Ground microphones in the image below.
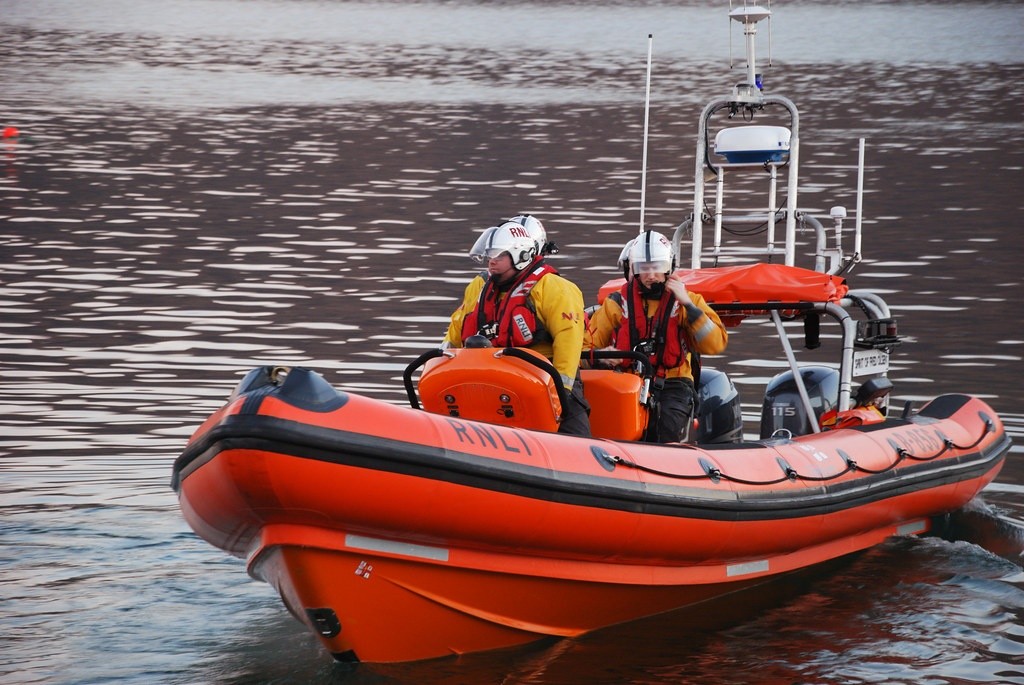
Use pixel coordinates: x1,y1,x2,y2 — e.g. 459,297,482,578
491,259,522,282
650,281,667,294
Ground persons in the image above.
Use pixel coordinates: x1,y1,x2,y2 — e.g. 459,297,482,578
581,229,729,445
440,213,592,440
616,238,638,283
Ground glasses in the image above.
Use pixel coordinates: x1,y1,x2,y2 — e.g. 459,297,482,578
486,256,502,260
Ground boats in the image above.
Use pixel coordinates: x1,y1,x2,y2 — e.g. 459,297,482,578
172,0,1013,662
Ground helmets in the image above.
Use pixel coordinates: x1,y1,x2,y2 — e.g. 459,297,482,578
621,231,676,275
485,215,548,270
